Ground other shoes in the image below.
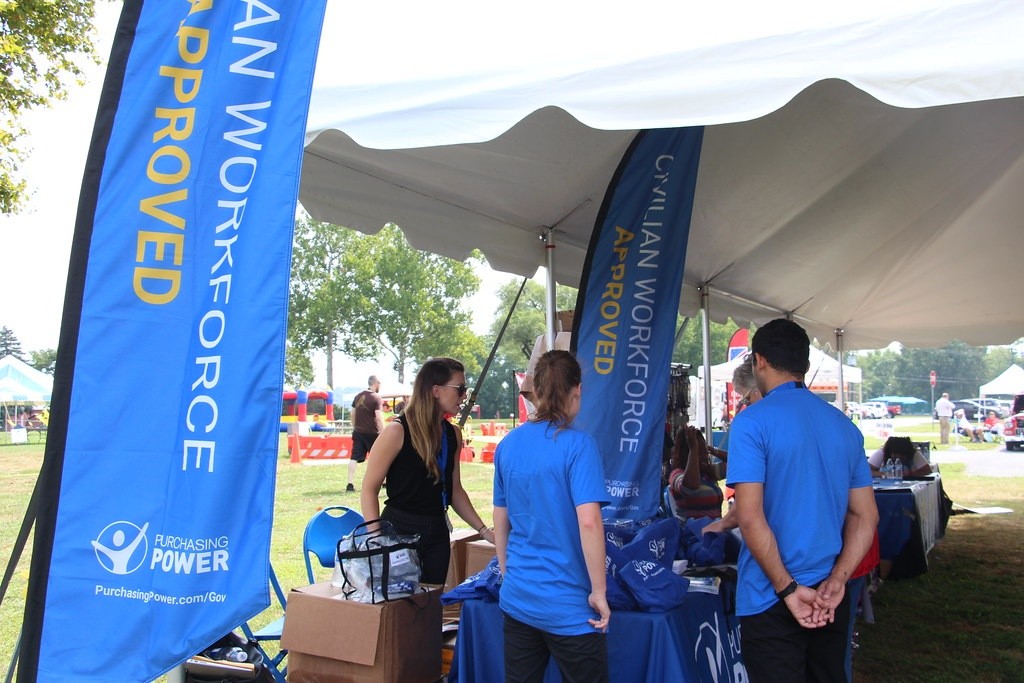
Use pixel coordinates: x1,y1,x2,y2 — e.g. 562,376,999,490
383,483,386,488
346,483,353,491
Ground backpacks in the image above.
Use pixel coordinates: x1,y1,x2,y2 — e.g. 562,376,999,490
664,485,686,522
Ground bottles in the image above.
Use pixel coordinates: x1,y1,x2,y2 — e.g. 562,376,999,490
885,458,894,478
204,647,248,664
894,458,903,480
332,535,352,587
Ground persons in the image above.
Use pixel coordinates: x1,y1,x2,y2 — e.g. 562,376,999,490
668,318,879,683
310,414,326,431
935,393,956,444
984,411,1002,429
361,358,495,593
954,409,985,443
492,348,612,683
21,410,36,427
346,374,387,491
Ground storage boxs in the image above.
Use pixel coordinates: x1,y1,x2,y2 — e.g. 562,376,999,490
442,528,496,620
280,582,445,682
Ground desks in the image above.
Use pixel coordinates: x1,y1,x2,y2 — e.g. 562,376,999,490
445,578,751,683
872,472,943,579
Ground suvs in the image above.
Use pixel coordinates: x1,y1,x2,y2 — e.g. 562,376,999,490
828,401,901,420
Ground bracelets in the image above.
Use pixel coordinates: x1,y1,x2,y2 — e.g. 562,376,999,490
478,525,486,532
774,578,799,601
480,528,489,537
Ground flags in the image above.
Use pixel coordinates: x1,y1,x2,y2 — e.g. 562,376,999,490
15,0,325,682
568,126,705,521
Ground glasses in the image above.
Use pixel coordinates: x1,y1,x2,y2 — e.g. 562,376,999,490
445,384,468,397
738,388,751,405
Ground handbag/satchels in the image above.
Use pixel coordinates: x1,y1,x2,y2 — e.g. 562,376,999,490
184,632,275,683
331,518,424,604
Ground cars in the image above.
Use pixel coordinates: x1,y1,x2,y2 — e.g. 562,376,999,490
1003,391,1024,451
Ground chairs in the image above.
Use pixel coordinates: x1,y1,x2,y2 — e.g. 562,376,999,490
303,505,366,584
664,484,685,521
242,562,288,683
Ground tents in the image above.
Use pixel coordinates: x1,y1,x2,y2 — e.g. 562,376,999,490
977,363,1024,427
295,0,1024,448
695,342,862,433
0,353,54,443
